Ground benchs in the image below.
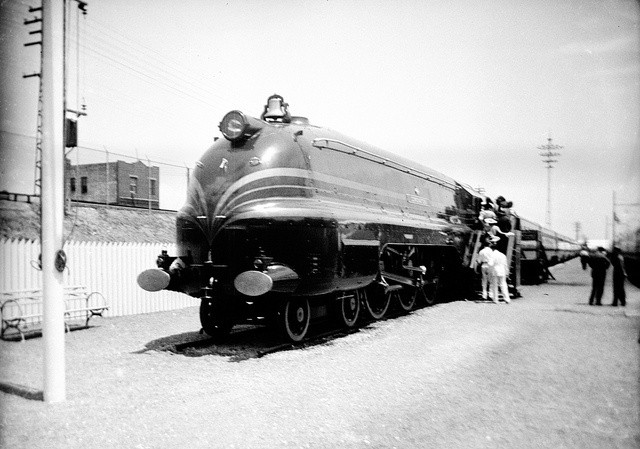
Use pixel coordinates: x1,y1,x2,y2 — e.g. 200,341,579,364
0,286,109,343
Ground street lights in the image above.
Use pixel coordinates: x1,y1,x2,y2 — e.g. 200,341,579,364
536,137,565,230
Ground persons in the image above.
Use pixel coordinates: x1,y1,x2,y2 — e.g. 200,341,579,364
475,197,512,248
478,243,493,300
588,247,611,305
489,246,511,304
580,247,589,269
611,246,627,305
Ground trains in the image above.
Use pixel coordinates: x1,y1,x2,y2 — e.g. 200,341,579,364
136,94,583,346
